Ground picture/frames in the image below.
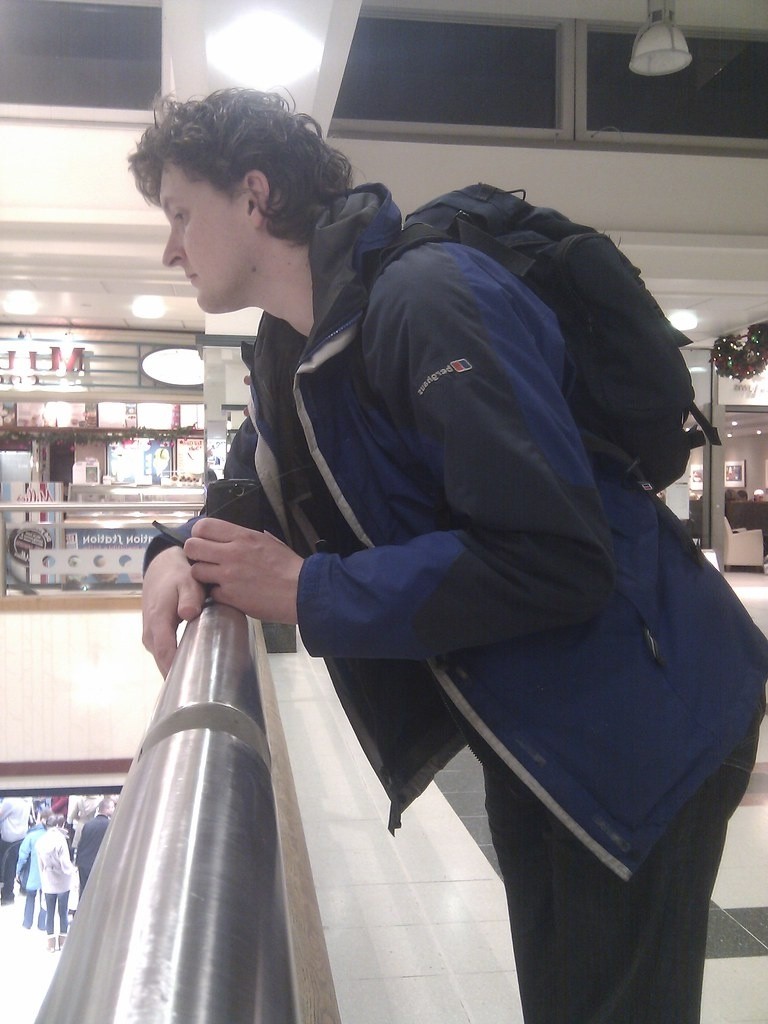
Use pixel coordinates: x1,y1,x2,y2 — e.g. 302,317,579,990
725,460,745,488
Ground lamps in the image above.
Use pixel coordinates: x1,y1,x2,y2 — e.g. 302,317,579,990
628,0,692,77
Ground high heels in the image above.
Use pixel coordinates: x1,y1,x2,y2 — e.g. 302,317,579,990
58,936,66,951
49,937,56,953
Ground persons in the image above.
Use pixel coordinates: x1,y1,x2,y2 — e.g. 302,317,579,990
129,88,768,1024
690,489,765,502
1,794,121,953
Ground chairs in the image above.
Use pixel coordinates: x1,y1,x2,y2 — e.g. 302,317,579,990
723,516,764,572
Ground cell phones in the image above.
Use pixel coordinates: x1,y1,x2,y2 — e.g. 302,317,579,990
205,478,264,533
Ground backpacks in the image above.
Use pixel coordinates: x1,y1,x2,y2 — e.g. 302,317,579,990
360,181,721,494
